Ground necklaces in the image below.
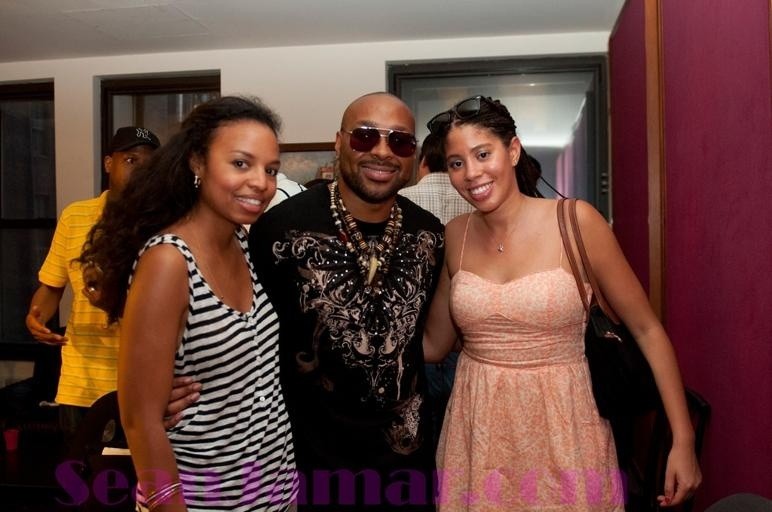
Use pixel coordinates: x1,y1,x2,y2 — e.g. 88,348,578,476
326,180,403,295
474,203,523,255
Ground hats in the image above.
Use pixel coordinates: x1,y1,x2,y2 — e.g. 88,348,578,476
109,126,161,154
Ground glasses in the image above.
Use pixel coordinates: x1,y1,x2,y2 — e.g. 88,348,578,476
341,127,418,157
427,94,485,135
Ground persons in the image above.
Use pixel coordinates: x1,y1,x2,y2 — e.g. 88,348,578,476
24,126,162,410
242,171,310,235
421,92,705,512
161,89,446,512
67,90,301,512
524,153,545,198
397,132,476,225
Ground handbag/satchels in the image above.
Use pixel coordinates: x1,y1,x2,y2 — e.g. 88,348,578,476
556,196,711,509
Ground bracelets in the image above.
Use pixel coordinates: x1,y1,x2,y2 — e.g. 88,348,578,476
143,482,183,511
